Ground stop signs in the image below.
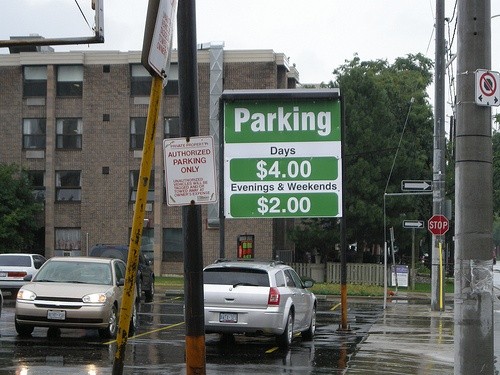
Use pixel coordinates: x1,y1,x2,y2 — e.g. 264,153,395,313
428,215,450,236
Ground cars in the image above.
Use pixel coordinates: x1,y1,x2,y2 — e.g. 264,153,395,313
14,256,139,340
0,253,48,289
183,258,318,349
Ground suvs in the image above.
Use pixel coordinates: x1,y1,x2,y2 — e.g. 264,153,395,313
88,242,155,300
